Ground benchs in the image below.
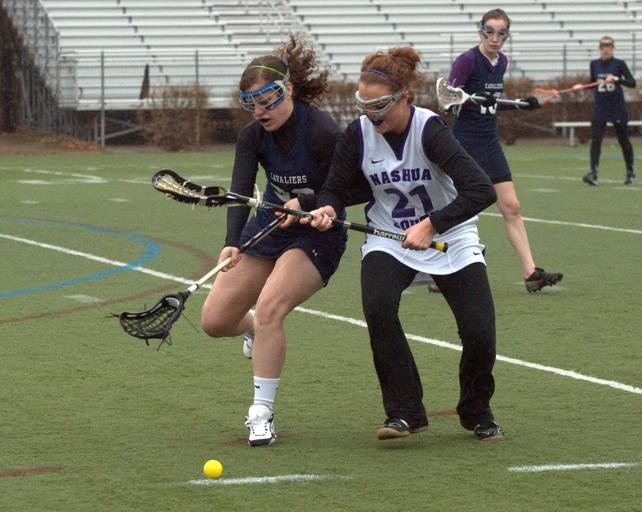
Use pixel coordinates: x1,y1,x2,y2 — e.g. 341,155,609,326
550,120,642,147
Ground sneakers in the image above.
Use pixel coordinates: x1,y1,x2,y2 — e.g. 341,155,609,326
624,174,635,186
244,404,277,447
428,284,439,293
474,422,504,441
243,308,255,360
376,416,429,439
526,268,563,292
583,172,600,185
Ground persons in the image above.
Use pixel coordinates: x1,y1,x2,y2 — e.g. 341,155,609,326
299,47,504,440
202,32,348,447
573,35,637,186
428,9,563,294
